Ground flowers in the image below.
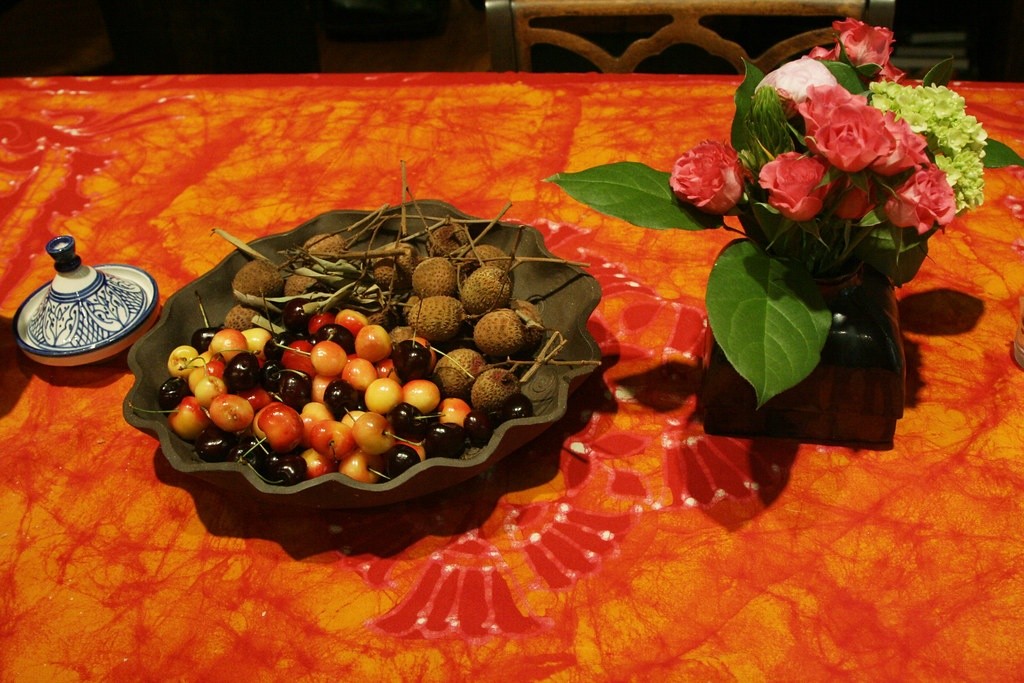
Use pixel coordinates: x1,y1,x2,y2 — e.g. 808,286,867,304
539,15,1024,410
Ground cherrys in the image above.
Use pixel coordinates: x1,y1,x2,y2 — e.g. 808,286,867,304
127,285,534,487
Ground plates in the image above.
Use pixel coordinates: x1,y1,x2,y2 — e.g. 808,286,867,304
11,263,161,366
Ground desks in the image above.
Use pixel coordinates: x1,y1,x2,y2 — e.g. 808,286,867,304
1,72,1024,683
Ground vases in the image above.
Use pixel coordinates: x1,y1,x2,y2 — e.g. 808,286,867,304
701,258,907,443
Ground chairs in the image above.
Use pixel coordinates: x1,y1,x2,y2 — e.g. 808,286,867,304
484,0,895,74
